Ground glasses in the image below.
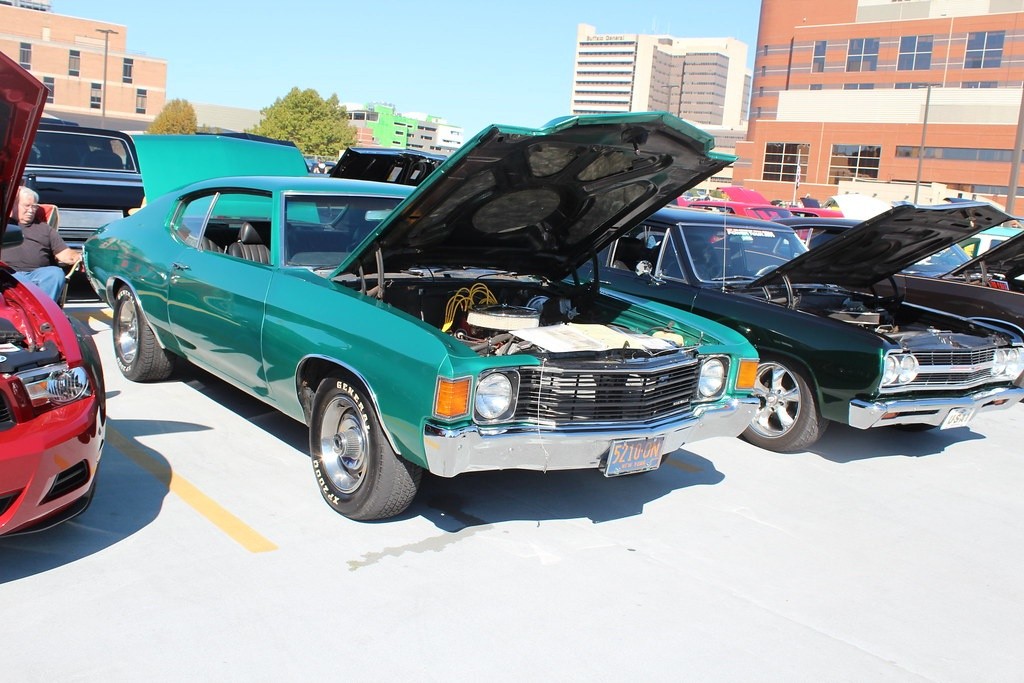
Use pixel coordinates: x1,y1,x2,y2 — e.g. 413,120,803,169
15,202,40,211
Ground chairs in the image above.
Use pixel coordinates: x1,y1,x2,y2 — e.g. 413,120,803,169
346,219,382,252
81,149,123,169
614,237,645,273
229,221,303,268
36,203,83,309
670,235,711,280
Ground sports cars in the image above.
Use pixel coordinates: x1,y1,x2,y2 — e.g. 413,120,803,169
79,110,765,522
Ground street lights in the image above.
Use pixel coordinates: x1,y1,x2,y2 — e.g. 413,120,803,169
96,28,120,117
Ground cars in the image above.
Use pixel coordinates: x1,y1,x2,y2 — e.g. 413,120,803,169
0,52,109,534
3,116,1024,455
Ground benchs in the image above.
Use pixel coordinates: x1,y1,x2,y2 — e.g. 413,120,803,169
186,227,352,257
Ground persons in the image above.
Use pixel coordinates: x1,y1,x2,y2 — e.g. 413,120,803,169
1,186,84,303
